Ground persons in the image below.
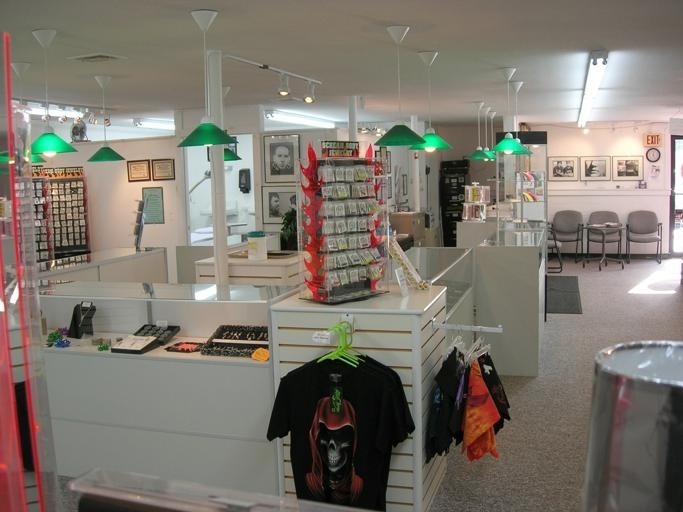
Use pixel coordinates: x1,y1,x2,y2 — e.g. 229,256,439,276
271,145,294,175
563,161,573,176
269,192,285,218
553,160,563,177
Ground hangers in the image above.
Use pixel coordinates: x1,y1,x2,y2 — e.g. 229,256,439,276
312,317,365,369
442,317,494,369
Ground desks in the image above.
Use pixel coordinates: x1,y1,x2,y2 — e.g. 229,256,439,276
580,222,627,271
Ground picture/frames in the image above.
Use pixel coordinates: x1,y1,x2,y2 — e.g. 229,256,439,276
149,157,176,182
546,155,579,182
610,155,642,182
373,146,407,203
261,132,303,184
259,184,298,225
124,159,151,182
579,155,611,181
140,186,165,226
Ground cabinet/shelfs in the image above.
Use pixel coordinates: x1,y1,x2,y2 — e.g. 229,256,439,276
404,247,476,354
0,244,168,298
12,278,308,512
473,228,547,378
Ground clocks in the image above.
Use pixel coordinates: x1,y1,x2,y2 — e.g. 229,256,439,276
646,148,659,163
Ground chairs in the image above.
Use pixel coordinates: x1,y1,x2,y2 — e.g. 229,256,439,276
547,210,584,263
622,209,663,265
523,219,564,274
584,209,625,265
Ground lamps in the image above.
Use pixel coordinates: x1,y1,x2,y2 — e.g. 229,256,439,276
372,25,532,164
221,54,322,106
573,48,611,130
204,86,242,165
0,27,130,176
172,9,239,151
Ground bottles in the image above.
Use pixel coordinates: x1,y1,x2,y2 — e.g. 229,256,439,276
247,231,267,260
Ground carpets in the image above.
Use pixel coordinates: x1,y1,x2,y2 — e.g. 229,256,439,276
543,275,583,316
